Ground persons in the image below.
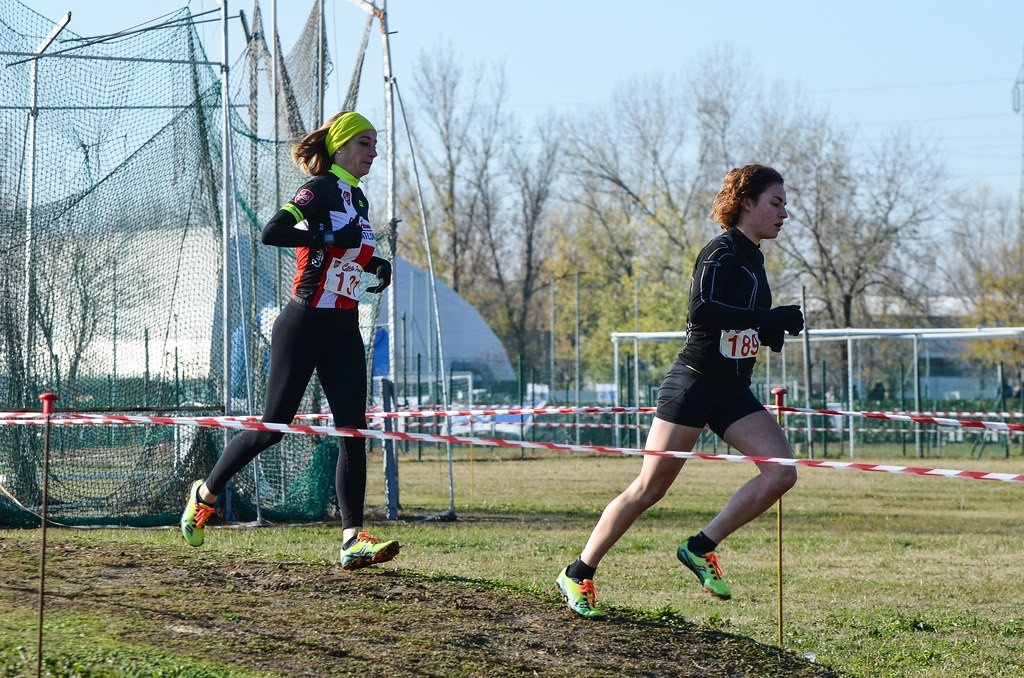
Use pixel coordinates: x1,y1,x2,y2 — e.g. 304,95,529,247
181,109,399,571
555,163,804,619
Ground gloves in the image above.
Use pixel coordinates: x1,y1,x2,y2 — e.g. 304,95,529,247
363,256,392,295
323,213,363,249
756,321,785,353
770,305,804,336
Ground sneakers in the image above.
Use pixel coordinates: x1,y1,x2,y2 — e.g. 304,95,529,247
676,536,731,601
179,478,217,548
554,565,602,619
340,529,400,569
